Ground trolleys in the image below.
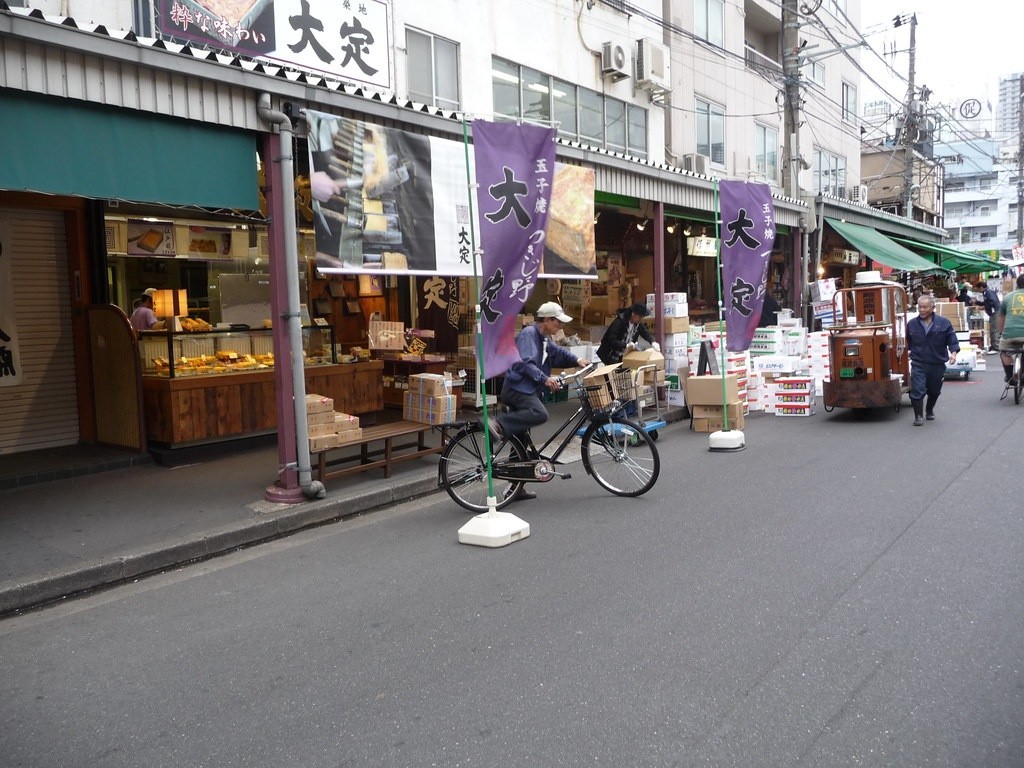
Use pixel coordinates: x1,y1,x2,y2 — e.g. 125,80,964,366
576,364,667,447
943,307,970,382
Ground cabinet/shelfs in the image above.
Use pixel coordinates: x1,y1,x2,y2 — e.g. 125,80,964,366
377,357,464,411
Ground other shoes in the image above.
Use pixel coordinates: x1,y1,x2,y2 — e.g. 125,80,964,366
487,419,502,442
505,488,536,500
987,350,998,354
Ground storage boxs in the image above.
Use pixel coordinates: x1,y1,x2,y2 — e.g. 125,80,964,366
457,368,490,393
458,346,476,370
292,393,333,414
309,434,339,450
337,428,362,444
307,410,335,425
403,406,457,424
335,411,359,432
409,373,453,397
404,389,457,411
308,422,335,437
514,251,991,433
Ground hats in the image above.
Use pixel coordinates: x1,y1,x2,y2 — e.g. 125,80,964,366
631,303,650,316
143,288,157,298
962,282,973,287
537,301,573,323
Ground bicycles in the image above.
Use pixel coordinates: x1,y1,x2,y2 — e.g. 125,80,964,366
436,363,661,514
1005,341,1024,405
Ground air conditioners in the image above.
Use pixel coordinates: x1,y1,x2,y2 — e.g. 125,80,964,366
635,38,671,90
601,40,632,78
682,153,710,176
852,184,867,205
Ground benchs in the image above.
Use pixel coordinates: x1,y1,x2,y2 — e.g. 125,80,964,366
311,420,449,484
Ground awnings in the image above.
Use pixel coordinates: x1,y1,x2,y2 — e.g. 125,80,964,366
823,217,1008,277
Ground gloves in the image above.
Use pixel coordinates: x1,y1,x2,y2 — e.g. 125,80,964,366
626,342,635,348
652,342,660,351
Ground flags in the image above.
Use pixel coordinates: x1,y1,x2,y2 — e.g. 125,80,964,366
471,118,558,385
719,178,778,353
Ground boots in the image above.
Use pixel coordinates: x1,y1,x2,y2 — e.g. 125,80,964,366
1004,364,1013,384
910,398,923,425
925,397,937,419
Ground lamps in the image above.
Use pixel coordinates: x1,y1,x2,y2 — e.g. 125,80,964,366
594,210,602,224
683,221,692,236
637,202,651,231
667,217,680,234
701,222,707,238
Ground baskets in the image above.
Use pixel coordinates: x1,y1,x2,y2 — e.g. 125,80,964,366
576,367,633,420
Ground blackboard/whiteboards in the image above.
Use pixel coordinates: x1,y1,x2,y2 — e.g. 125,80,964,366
696,340,720,375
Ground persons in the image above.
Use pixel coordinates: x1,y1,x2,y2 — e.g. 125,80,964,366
998,274,1024,387
955,282,1000,355
478,302,592,502
129,288,166,340
906,295,960,426
306,111,340,231
596,301,660,365
